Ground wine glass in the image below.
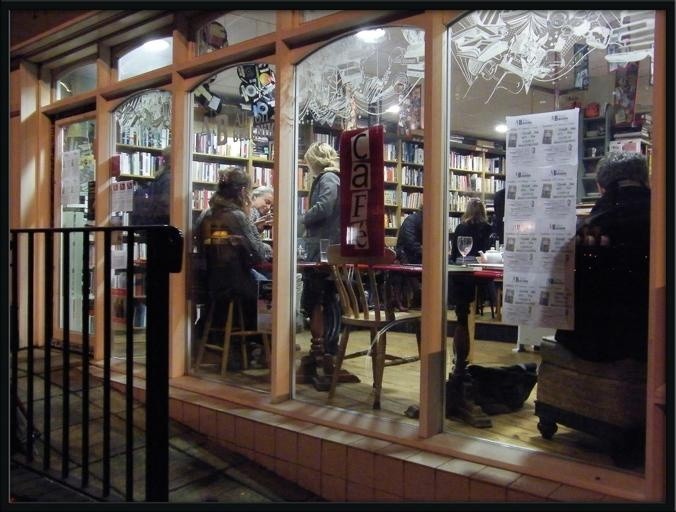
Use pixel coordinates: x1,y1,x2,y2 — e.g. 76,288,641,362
456,236,473,267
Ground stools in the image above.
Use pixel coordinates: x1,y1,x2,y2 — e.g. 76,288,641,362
533,334,648,476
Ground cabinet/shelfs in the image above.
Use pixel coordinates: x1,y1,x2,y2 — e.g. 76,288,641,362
578,100,652,219
61,91,504,250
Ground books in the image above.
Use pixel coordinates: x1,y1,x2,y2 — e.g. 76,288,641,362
297,132,506,234
77,120,276,290
573,113,652,217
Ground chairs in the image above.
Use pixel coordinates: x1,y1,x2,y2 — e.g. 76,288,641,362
193,242,504,411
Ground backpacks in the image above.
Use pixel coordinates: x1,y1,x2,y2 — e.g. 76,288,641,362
129,174,174,234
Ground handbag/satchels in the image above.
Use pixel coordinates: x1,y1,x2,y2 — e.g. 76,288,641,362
466,361,539,416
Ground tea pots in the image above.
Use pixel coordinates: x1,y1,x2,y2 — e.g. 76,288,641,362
477,246,503,264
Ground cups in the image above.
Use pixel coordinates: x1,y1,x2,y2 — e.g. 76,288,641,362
319,238,331,262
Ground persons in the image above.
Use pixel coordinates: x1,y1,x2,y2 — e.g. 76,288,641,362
191,165,271,369
392,187,506,309
299,140,344,357
544,152,651,366
